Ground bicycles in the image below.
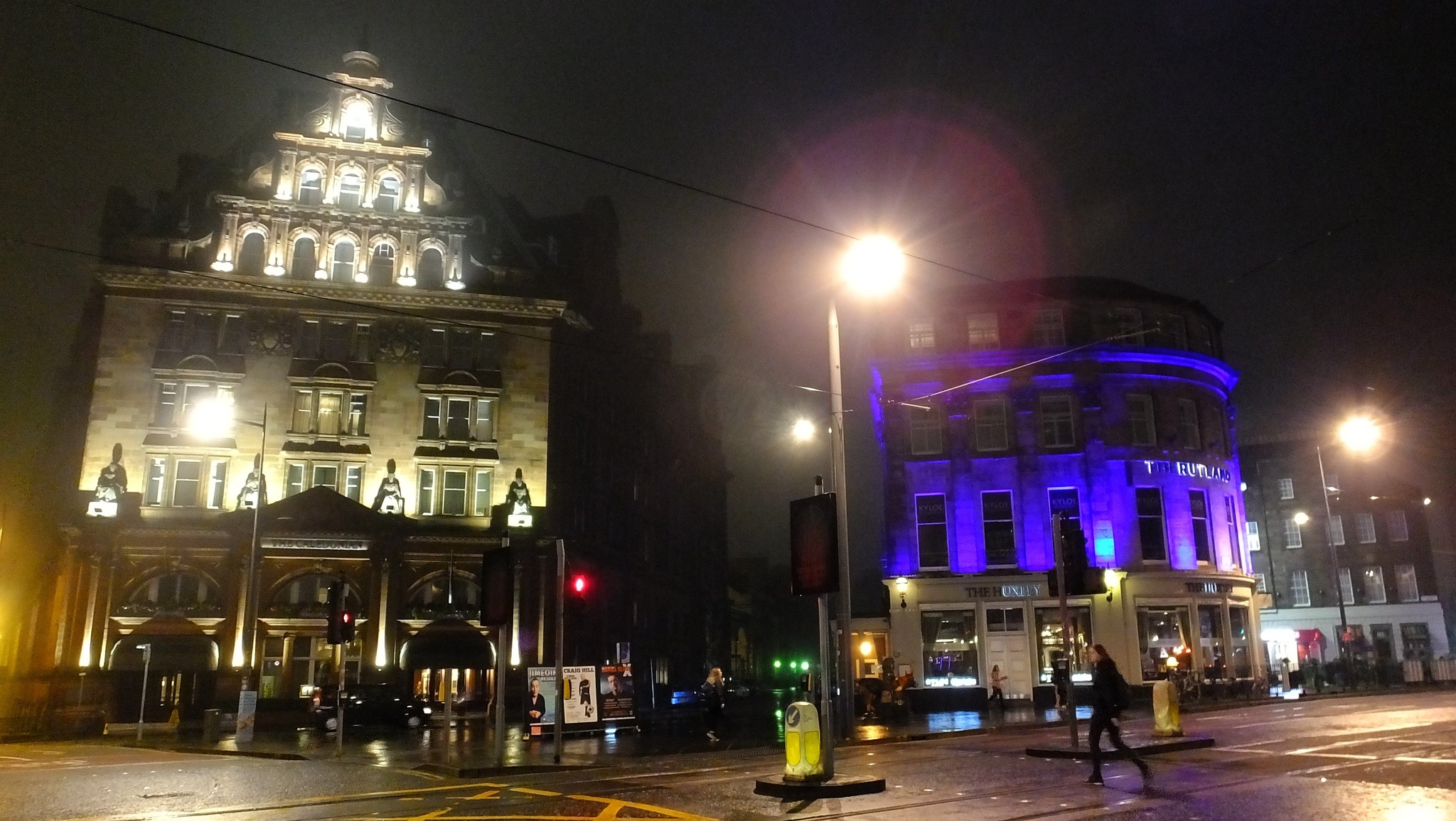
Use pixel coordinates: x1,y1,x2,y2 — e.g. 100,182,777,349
1169,669,1202,707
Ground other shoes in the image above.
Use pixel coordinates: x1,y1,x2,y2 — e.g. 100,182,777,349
1141,764,1154,789
706,731,720,742
1083,774,1104,785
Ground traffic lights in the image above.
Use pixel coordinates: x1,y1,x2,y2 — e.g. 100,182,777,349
341,610,356,642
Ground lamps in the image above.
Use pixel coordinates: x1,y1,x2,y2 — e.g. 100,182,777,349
895,577,908,608
1104,563,1119,602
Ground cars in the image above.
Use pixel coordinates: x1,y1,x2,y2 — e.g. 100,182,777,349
305,683,432,734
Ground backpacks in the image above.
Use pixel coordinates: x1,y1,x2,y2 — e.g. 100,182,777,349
696,687,716,713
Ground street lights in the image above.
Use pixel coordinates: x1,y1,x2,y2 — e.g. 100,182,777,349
1316,414,1383,692
824,232,908,743
190,395,268,687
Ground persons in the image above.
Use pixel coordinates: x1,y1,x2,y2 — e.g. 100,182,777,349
701,667,725,742
1082,644,1152,788
579,673,591,719
602,675,634,699
528,679,546,724
988,665,1007,701
